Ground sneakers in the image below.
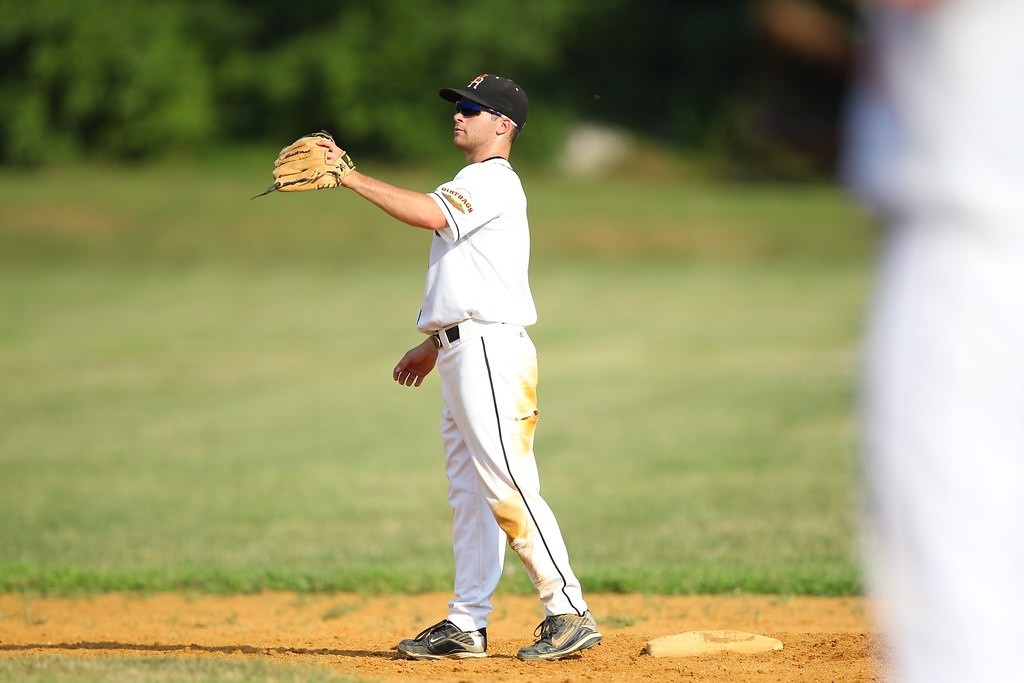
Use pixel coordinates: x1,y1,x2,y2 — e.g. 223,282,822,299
398,619,489,659
517,609,603,661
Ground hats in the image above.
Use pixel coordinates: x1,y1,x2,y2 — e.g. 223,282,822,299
439,73,528,131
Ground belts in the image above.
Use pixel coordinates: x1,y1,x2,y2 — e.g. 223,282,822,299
433,322,459,348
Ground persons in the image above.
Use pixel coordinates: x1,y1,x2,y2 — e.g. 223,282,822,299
847,0,1024,682
315,72,602,660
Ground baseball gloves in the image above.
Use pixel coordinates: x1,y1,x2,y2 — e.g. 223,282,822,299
272,130,357,193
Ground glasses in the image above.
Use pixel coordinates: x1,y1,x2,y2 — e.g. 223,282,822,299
456,101,518,127
